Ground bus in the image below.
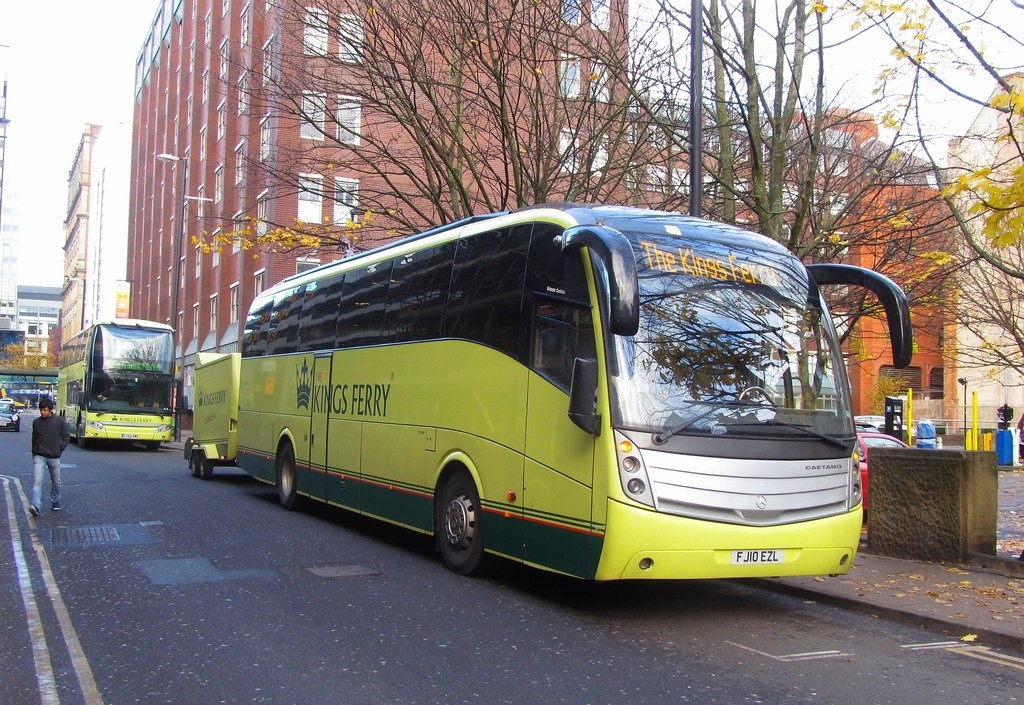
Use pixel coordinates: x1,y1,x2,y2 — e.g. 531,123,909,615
55,318,184,451
234,199,915,587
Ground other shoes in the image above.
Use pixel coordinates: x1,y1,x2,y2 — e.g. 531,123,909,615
29,504,40,516
52,503,61,510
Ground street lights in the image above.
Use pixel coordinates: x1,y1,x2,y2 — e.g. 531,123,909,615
958,377,967,450
155,153,189,333
68,276,86,331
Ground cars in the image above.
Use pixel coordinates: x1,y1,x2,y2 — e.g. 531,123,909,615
0,398,24,431
855,431,911,510
854,413,916,436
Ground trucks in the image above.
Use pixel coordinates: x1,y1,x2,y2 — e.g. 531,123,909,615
184,351,241,480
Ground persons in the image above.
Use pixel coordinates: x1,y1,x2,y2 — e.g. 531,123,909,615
28,399,69,516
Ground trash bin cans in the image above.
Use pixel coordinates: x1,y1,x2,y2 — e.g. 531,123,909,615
996,429,1012,465
